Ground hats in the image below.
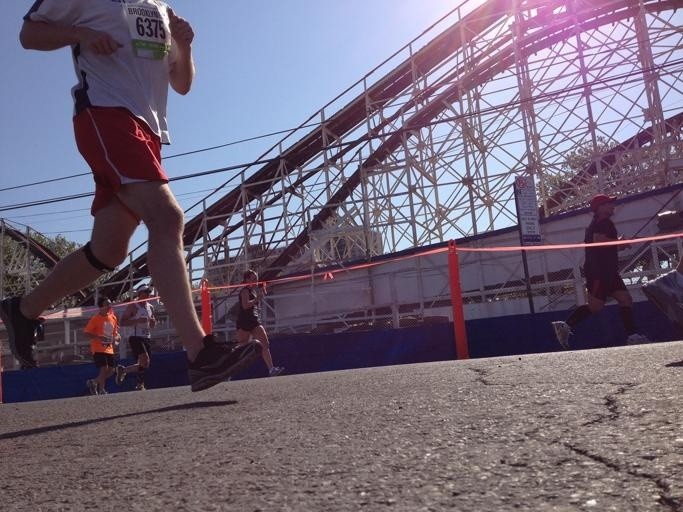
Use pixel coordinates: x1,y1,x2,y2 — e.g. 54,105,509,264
136,284,151,292
591,194,616,212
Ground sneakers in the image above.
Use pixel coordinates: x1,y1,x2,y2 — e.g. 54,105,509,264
268,367,284,376
87,379,107,395
551,321,576,349
184,333,263,391
136,385,145,391
115,365,126,384
627,332,653,345
0,297,44,367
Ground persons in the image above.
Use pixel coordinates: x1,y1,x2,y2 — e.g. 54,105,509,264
640,256,682,329
0,1,260,394
551,194,651,350
113,283,156,392
235,268,285,378
81,296,121,396
